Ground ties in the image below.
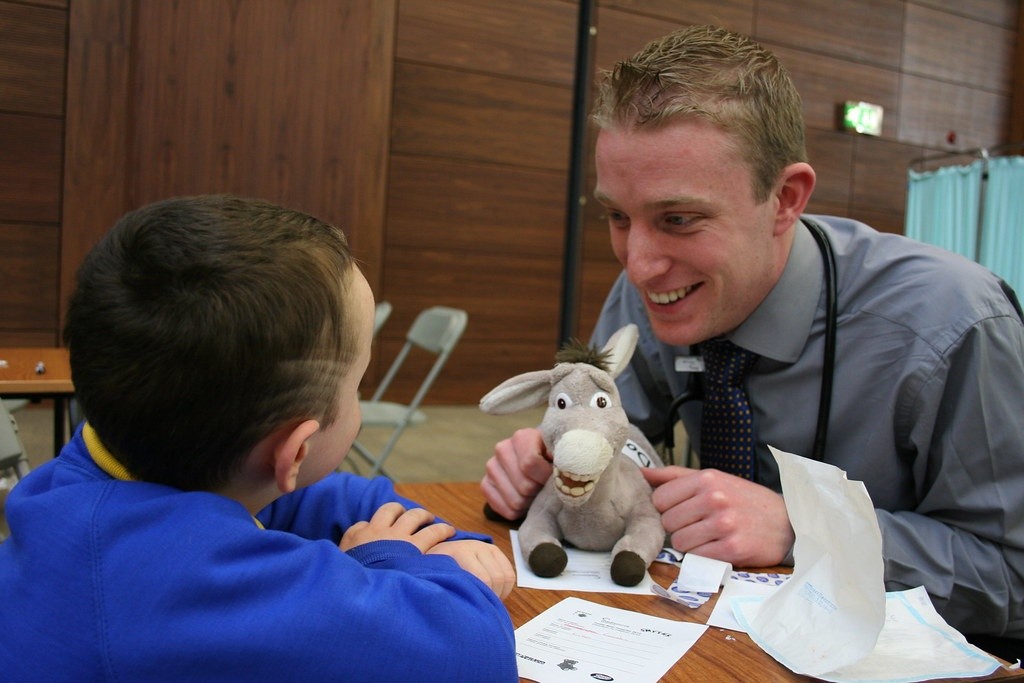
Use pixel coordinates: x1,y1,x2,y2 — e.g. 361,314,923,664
695,342,761,481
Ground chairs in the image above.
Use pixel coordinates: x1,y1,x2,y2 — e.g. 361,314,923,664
343,304,468,484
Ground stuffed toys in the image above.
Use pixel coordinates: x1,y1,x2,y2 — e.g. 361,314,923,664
478,322,666,587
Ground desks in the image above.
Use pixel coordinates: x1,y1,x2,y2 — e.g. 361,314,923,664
0,348,75,458
389,478,1023,683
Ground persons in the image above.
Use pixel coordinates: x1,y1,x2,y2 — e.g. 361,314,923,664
480,24,1024,669
0,192,519,683
